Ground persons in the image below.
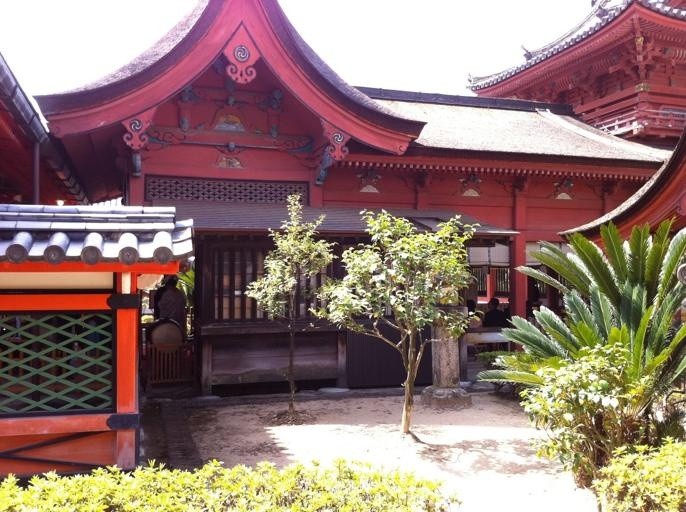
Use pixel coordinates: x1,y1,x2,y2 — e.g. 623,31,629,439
484,297,505,355
467,299,480,318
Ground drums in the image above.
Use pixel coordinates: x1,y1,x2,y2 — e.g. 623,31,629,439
150,318,185,354
